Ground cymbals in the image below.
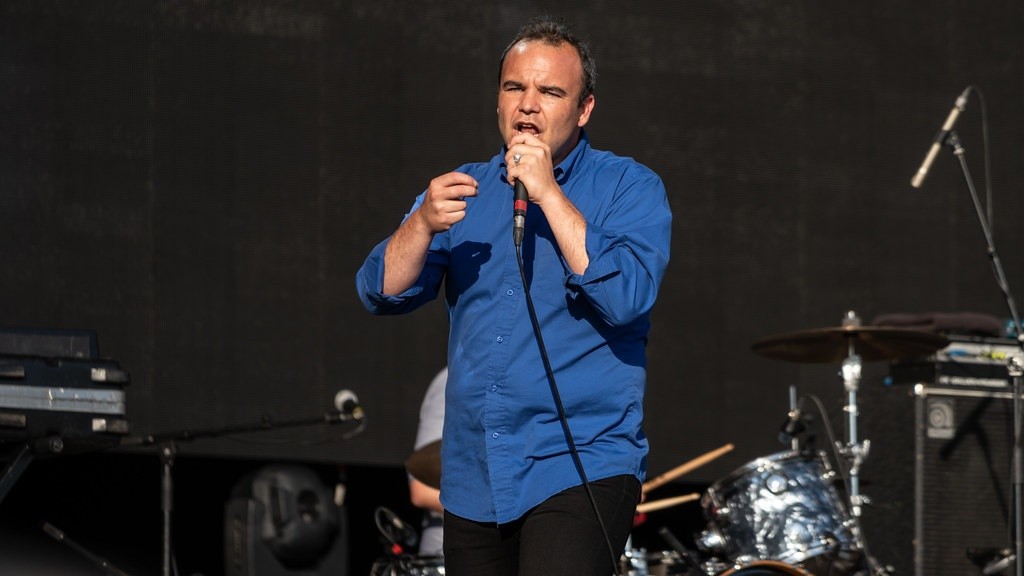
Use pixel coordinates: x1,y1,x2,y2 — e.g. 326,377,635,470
407,438,443,489
758,325,951,367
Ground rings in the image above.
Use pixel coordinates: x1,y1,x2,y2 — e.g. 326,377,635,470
513,153,522,166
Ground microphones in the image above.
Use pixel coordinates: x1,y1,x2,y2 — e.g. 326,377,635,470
385,509,417,546
776,408,803,446
910,94,969,189
512,177,528,246
334,388,365,420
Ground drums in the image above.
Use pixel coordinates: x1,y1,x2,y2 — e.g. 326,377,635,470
708,557,818,576
372,553,444,576
698,449,860,570
632,548,707,576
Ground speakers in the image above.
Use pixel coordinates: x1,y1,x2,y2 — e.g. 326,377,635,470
895,383,1024,576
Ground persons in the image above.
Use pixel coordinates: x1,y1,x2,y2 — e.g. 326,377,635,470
354,16,672,576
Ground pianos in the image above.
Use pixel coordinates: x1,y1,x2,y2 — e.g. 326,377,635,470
0,330,130,449
887,337,1024,389
